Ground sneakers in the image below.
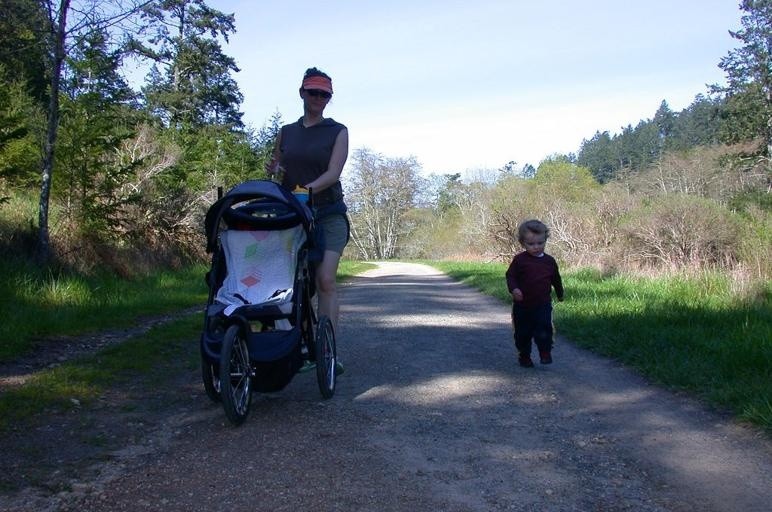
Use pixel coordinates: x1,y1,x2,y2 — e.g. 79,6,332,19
299,349,344,377
517,353,553,368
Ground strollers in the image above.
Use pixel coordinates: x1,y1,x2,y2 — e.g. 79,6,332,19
199,180,338,425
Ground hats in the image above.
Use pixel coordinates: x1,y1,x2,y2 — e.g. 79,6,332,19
303,76,334,94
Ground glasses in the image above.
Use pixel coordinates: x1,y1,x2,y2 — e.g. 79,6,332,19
305,89,330,99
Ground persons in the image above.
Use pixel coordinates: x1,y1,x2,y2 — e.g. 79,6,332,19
505,217,566,368
266,68,351,378
